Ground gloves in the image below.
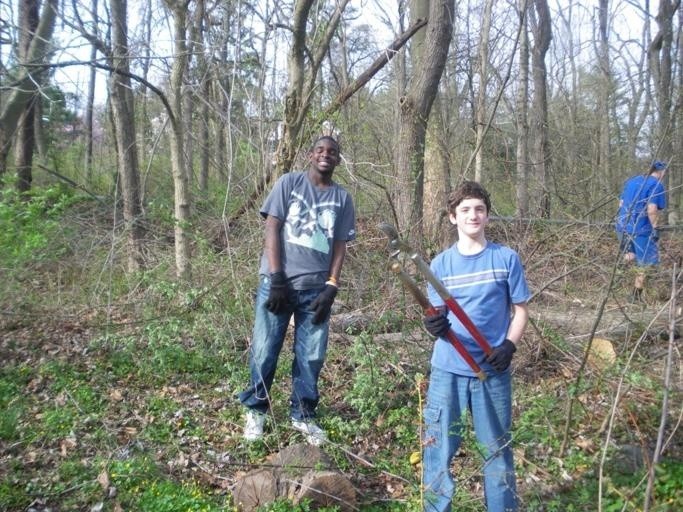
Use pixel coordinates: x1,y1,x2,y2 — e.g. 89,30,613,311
482,338,517,371
651,230,660,243
311,284,337,324
423,313,452,336
264,272,290,315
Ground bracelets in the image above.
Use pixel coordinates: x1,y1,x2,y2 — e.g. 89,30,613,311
325,277,338,288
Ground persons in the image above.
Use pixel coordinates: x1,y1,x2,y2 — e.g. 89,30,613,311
615,162,668,307
422,180,530,512
238,136,354,447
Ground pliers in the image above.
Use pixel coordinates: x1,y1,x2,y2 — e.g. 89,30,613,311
372,222,504,382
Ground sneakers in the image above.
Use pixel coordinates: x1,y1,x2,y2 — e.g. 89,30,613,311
627,294,647,305
242,410,263,441
291,417,324,447
